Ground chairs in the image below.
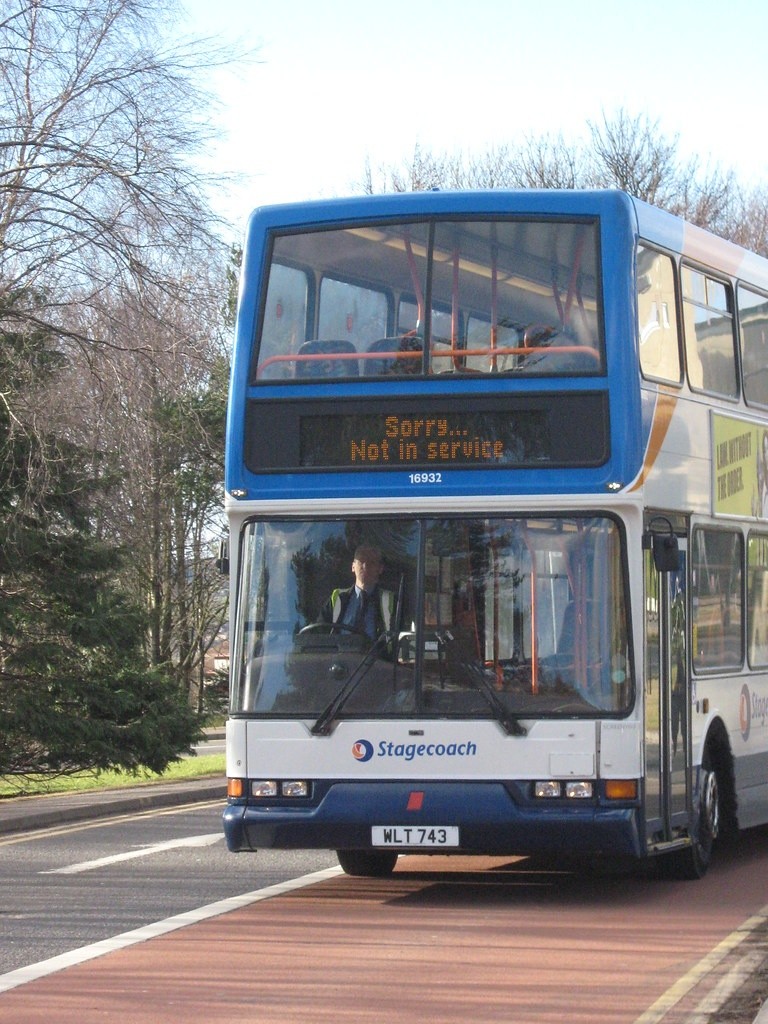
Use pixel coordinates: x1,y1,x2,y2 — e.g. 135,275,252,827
295,336,427,377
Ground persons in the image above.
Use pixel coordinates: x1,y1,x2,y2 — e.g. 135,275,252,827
307,541,398,658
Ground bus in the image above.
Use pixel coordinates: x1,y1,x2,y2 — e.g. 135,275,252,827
213,192,768,888
